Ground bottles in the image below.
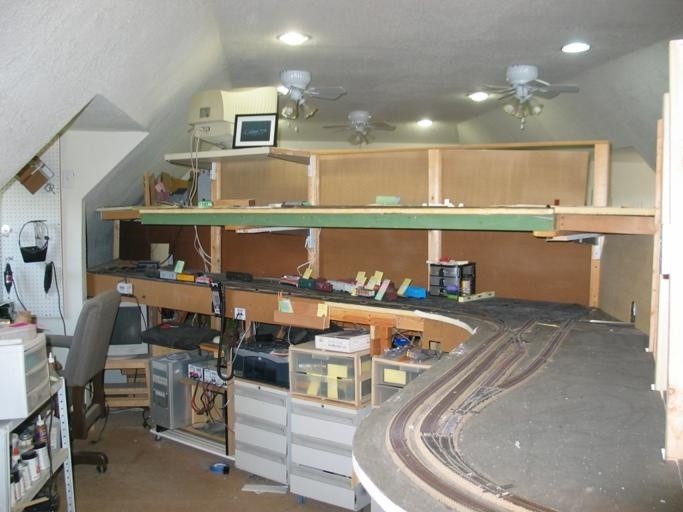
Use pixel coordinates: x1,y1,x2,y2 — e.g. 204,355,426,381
9,409,60,508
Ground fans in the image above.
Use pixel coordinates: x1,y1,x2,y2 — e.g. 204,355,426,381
275,67,398,149
484,64,582,124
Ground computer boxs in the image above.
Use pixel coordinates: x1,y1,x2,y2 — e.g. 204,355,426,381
150,349,214,429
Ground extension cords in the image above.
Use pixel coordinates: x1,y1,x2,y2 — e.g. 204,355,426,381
138,262,158,271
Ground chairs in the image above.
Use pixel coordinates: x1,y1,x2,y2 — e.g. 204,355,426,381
26,290,122,476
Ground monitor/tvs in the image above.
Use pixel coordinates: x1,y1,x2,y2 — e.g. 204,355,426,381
188,85,278,149
105,302,148,360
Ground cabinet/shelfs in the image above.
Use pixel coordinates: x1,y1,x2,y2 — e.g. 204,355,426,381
1,370,82,510
85,141,613,512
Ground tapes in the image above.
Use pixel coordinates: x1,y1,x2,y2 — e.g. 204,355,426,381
209,462,230,474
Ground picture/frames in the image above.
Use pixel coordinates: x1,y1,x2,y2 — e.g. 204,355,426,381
230,111,279,149
